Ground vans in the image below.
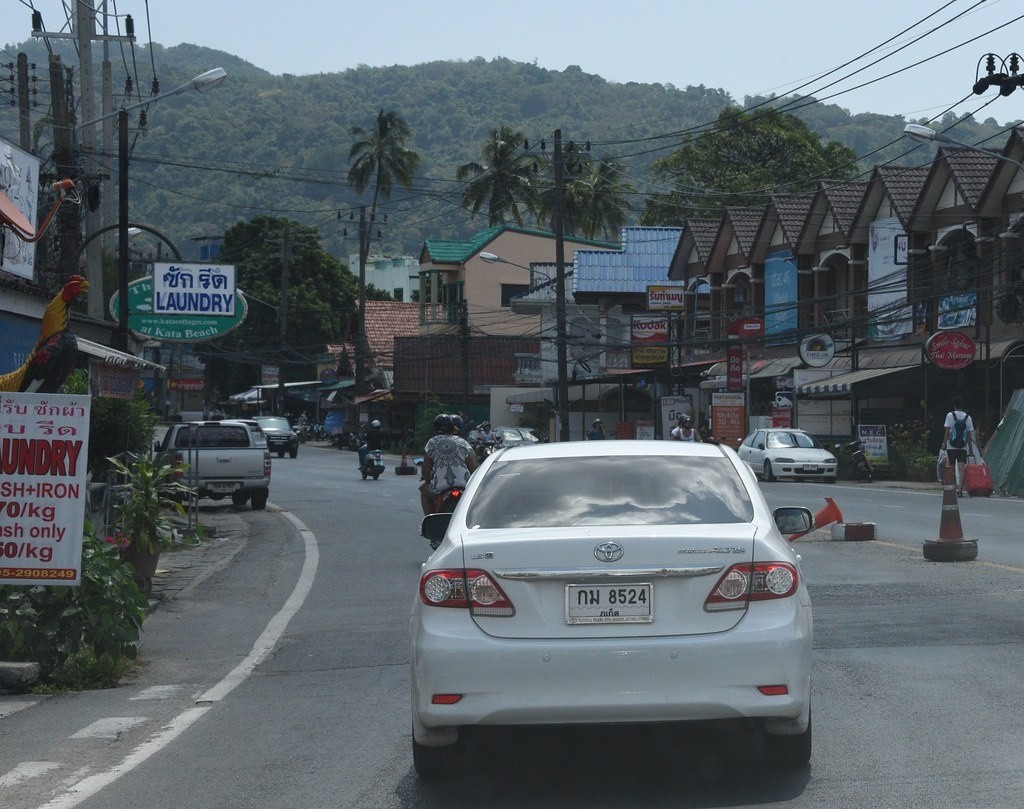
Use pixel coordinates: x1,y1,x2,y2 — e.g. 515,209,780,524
235,420,269,448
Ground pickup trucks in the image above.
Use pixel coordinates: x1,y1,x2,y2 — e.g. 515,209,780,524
151,420,271,509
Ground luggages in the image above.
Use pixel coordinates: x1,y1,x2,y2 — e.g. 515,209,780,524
966,454,993,497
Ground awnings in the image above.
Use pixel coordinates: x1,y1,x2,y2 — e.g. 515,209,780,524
71,334,167,375
250,380,323,424
794,367,926,437
318,379,369,398
507,358,730,442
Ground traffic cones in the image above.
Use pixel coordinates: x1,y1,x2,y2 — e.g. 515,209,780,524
787,497,844,542
400,448,408,467
924,466,978,543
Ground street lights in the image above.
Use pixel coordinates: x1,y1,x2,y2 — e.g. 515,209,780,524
478,250,571,442
53,66,228,290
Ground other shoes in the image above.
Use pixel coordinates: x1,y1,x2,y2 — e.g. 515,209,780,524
358,467,362,470
957,491,962,497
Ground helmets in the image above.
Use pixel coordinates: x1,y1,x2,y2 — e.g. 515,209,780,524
450,414,466,431
483,421,489,426
372,420,380,428
683,415,693,422
680,414,686,420
592,419,602,426
434,413,451,432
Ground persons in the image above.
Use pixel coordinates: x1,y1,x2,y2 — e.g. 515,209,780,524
671,414,703,443
587,418,610,440
476,421,498,456
418,413,478,515
357,419,385,470
941,397,974,497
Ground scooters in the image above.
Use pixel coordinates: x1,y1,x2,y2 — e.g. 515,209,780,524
469,435,503,465
350,433,386,480
413,457,466,549
292,419,366,451
835,433,875,484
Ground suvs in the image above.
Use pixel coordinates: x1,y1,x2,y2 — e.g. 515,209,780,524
251,416,299,459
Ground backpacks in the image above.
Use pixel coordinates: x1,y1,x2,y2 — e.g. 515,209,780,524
949,411,969,447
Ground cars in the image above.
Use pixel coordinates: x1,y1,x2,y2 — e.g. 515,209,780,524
737,427,838,484
409,439,817,779
229,388,264,408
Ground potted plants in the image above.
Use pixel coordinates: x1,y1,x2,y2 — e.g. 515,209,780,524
105,452,201,578
914,455,939,482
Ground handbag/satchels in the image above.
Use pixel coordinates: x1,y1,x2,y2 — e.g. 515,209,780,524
936,449,948,481
